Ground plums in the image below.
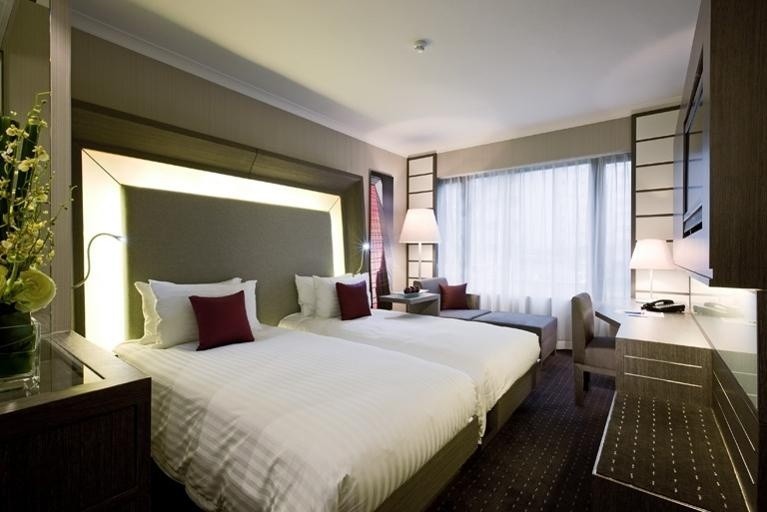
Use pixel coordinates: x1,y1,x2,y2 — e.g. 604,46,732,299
404,286,419,294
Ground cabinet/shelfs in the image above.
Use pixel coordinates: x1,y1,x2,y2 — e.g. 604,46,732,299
671,0,767,287
592,391,754,512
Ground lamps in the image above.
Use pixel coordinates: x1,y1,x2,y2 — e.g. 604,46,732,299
355,242,370,274
72,232,126,290
398,208,443,279
628,236,672,301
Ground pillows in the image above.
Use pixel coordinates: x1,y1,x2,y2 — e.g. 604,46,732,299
293,270,373,321
132,272,261,349
438,282,469,310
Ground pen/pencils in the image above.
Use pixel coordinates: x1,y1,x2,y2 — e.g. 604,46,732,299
628,315,648,317
625,311,641,314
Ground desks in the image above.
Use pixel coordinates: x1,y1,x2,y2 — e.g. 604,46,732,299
0,331,153,510
379,292,442,316
594,298,714,408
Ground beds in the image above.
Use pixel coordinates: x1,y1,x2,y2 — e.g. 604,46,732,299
278,307,540,447
115,324,484,511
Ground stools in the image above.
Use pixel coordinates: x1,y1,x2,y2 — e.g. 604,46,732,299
474,311,558,369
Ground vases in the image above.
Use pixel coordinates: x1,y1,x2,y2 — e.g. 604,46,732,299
0,317,41,390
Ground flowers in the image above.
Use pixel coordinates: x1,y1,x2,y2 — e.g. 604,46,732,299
0,88,80,312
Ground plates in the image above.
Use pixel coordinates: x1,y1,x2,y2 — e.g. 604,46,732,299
393,289,429,298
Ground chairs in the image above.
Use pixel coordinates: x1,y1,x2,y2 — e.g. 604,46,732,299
411,277,491,321
570,292,617,404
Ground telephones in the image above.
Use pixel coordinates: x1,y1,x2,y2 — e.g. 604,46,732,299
646,300,685,312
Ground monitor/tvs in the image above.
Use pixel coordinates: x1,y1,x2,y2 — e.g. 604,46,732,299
683,70,703,234
